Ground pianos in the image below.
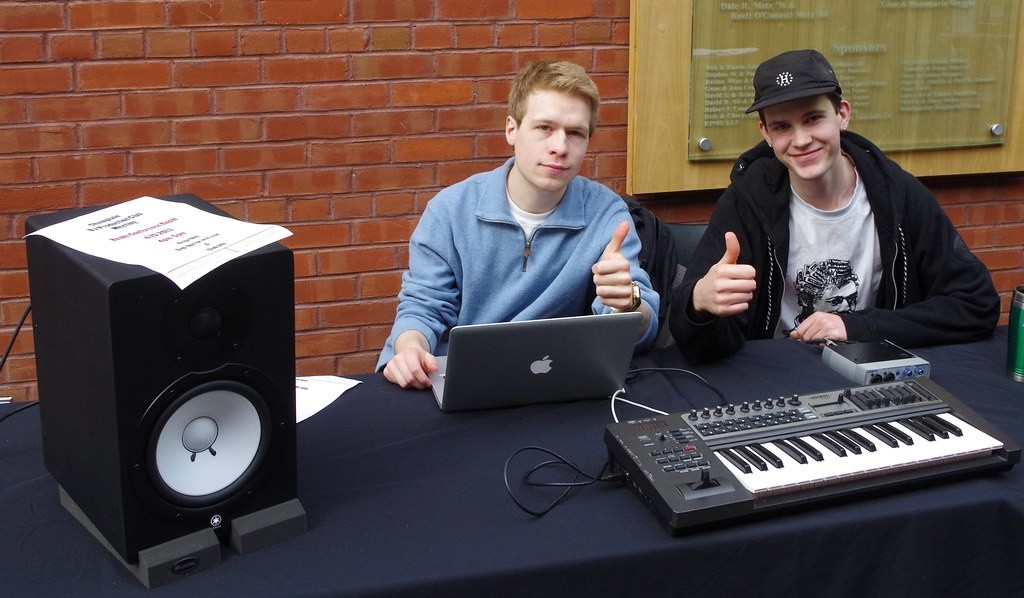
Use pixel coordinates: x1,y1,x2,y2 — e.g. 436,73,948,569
603,372,1024,540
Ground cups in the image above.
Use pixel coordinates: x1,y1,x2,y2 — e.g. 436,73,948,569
1005,285,1024,384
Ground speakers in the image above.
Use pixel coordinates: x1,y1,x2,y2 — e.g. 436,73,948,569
25,191,299,564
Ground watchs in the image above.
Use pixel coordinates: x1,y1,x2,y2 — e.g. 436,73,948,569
608,281,641,314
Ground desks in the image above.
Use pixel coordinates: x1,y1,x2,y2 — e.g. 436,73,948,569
0,325,1024,598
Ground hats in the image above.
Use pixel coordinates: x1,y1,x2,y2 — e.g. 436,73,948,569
744,49,842,113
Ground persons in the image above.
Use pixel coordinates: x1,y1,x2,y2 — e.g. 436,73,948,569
668,46,1001,368
375,60,662,392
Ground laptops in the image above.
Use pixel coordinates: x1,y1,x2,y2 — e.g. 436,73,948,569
426,311,644,410
